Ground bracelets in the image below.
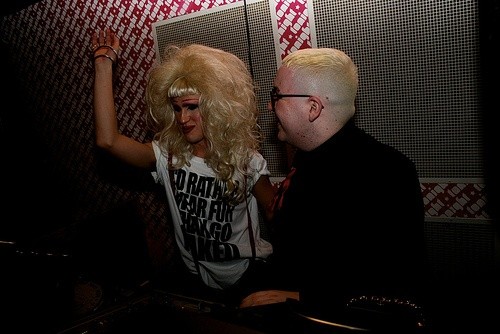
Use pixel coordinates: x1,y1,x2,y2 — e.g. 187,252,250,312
92,43,118,59
93,53,116,66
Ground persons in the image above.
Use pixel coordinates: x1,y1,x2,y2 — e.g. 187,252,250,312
237,47,427,333
91,28,280,334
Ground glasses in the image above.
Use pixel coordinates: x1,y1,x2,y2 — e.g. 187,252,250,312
269,91,324,110
